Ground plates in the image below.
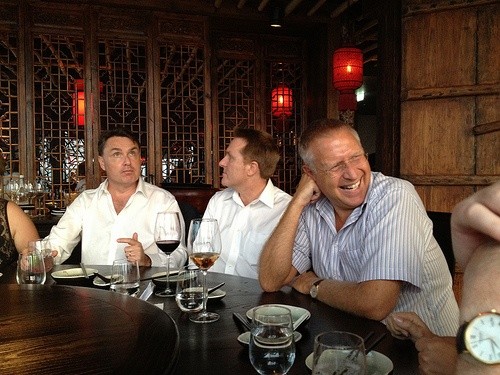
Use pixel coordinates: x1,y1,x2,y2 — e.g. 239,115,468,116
245,304,310,326
237,331,302,348
305,348,393,375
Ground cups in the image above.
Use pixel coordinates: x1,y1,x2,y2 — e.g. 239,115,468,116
176,265,208,313
110,259,140,298
312,330,366,375
28,237,53,272
249,305,295,375
16,252,46,284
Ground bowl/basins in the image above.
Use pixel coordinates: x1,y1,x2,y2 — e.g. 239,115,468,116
93,274,124,290
51,268,98,287
181,287,226,302
109,282,140,295
152,270,197,291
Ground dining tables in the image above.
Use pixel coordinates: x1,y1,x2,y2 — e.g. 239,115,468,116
0,265,423,375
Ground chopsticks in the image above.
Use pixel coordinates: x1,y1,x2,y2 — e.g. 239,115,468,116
234,312,253,331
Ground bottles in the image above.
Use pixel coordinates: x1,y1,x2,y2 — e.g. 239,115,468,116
18,175,25,188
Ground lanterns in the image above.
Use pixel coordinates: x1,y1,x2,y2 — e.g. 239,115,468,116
271,82,293,119
67,80,104,125
332,44,364,111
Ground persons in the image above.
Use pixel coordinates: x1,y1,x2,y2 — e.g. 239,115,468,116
386,180,500,374
258,118,460,336
20,128,187,273
0,151,39,266
75,162,86,191
187,129,293,281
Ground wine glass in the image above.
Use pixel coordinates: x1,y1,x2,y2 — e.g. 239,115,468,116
5,179,44,218
188,218,221,322
153,212,181,297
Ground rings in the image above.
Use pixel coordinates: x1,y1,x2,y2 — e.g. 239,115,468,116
129,251,131,256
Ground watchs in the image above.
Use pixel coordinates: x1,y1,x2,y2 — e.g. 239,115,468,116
456,309,500,365
309,279,325,298
